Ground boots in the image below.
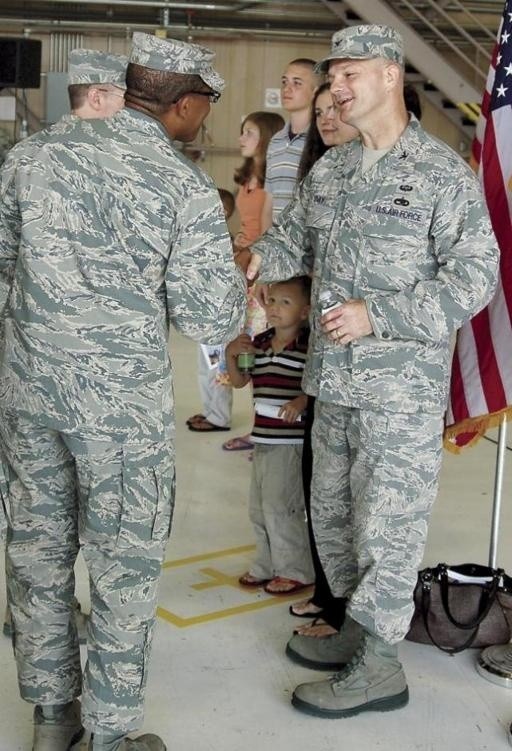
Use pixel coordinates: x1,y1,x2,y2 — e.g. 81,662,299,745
80,733,168,751
285,614,396,668
28,699,86,751
290,633,410,720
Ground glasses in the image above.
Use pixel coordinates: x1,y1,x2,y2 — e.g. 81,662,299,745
173,88,222,105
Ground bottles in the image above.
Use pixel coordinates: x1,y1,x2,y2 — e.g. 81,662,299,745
238,352,255,372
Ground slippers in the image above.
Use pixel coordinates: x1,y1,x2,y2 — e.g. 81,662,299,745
221,435,255,452
239,571,267,588
293,619,339,639
288,599,324,618
187,415,207,426
262,575,308,595
189,421,231,433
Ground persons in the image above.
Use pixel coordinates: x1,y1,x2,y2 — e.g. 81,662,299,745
185,189,234,432
285,79,424,635
0,29,250,751
4,49,130,656
224,274,317,595
221,109,285,461
296,81,334,183
240,20,500,716
258,57,320,231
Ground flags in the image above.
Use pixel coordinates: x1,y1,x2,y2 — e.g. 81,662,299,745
442,0,512,452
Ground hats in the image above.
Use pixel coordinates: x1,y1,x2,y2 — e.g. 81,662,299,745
67,48,129,90
128,31,226,92
311,22,407,76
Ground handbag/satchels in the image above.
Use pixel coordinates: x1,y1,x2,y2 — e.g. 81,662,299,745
401,562,511,654
